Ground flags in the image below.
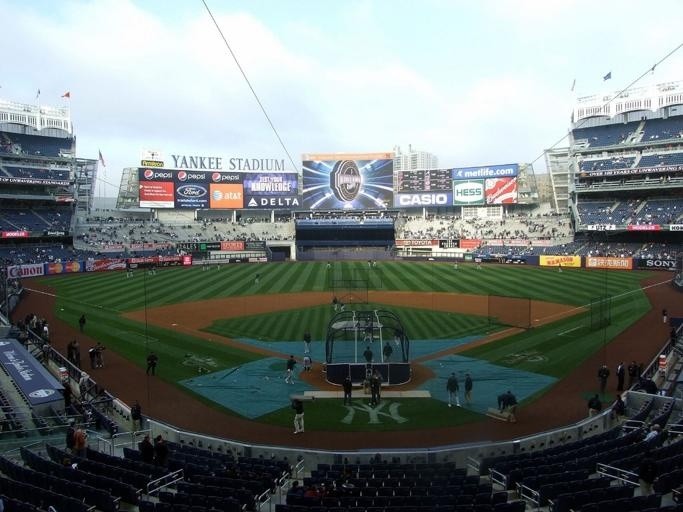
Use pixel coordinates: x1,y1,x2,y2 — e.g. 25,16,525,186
35,89,40,99
61,92,69,98
603,72,610,81
570,79,575,90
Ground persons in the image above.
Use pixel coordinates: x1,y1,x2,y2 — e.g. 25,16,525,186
280,291,403,435
367,258,376,267
253,273,260,287
558,263,563,272
675,271,680,282
446,372,461,408
477,260,481,271
14,311,179,473
496,394,509,414
506,390,520,423
297,212,395,220
268,452,401,497
586,360,662,445
462,373,473,407
396,127,683,260
1,126,292,277
637,450,658,498
661,307,668,324
455,258,458,270
670,271,675,288
327,261,331,269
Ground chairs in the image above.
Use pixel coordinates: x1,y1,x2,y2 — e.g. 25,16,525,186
0,386,683,512
